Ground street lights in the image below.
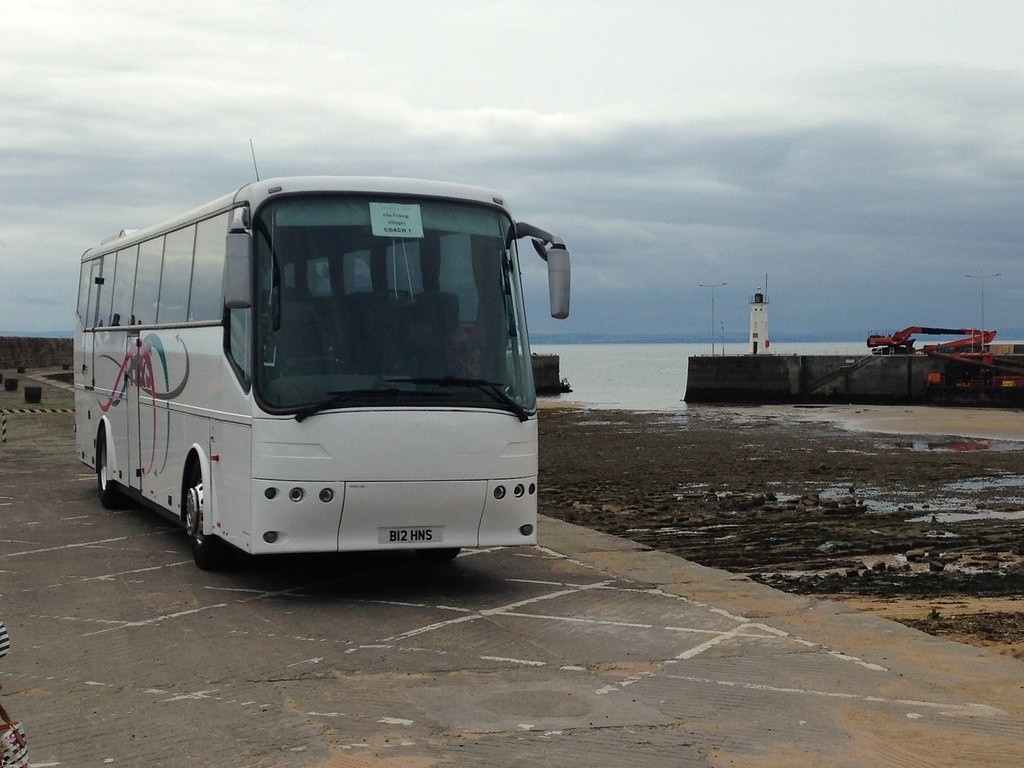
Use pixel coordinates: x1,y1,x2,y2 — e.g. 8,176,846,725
963,273,1001,353
698,282,728,356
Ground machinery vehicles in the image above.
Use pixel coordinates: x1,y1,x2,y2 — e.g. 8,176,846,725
867,325,996,355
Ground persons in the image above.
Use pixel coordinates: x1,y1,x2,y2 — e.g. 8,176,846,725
434,327,504,405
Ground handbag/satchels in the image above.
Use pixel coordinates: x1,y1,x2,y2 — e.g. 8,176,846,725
0,706,30,768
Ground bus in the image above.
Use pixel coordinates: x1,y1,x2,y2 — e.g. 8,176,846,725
74,176,571,571
